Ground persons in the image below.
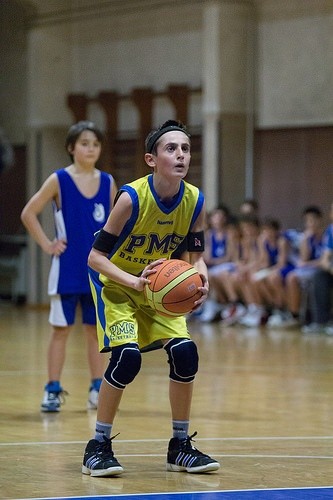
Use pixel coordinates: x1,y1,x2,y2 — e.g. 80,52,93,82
81,125,221,476
21,123,122,413
192,198,333,335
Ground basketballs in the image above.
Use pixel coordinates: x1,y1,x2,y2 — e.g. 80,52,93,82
143,258,203,318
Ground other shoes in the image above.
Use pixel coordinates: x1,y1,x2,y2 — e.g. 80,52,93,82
195,299,299,328
302,322,333,335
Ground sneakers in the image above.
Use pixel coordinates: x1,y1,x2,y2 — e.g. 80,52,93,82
87,386,103,410
40,381,64,413
80,439,124,477
166,437,220,474
